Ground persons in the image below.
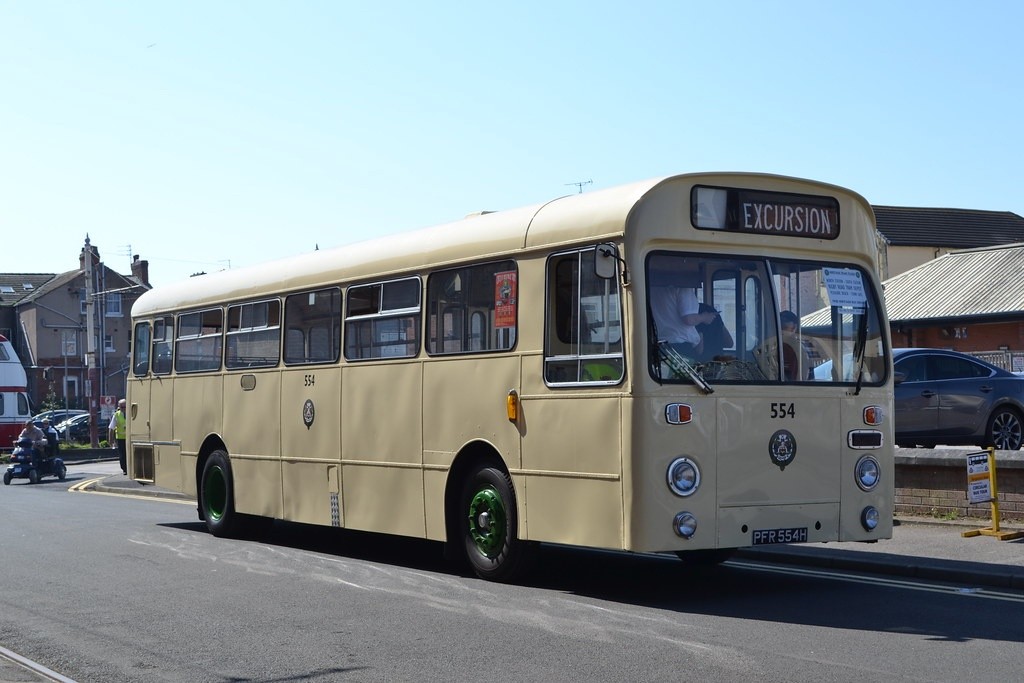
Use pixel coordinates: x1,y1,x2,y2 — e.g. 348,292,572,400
648,288,718,360
775,311,798,332
10,418,59,462
108,399,127,475
695,303,734,361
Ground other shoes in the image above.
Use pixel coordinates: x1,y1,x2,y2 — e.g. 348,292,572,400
123,472,127,475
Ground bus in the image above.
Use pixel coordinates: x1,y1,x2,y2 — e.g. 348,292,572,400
113,171,896,590
0,335,31,450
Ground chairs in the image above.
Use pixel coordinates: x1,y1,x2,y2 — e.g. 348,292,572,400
136,361,157,373
199,355,219,369
155,348,180,373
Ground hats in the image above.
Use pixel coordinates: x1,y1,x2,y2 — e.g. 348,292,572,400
779,311,798,323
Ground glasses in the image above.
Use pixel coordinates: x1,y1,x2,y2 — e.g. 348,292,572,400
43,423,48,425
121,407,126,408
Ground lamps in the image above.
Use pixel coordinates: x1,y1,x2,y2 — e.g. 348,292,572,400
941,327,956,336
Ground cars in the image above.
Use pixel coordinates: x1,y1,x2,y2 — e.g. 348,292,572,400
804,350,1023,452
31,409,112,450
